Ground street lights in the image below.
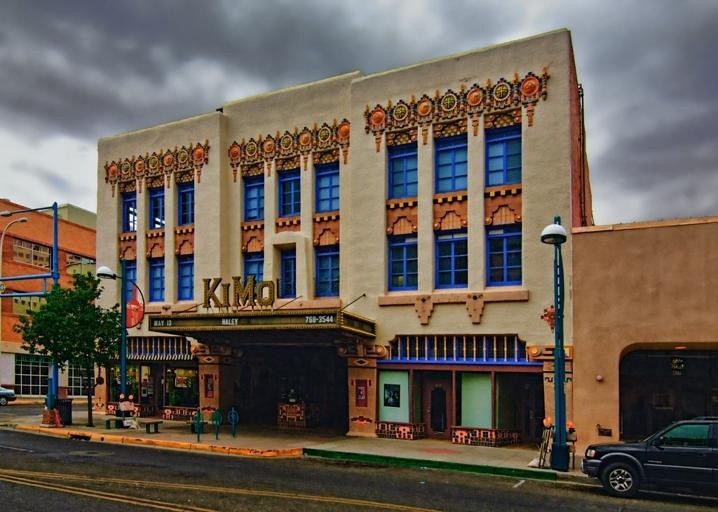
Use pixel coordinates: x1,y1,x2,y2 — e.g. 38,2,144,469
539,215,569,472
96,266,145,429
0,202,59,428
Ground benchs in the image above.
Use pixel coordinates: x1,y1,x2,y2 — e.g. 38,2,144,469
101,414,164,433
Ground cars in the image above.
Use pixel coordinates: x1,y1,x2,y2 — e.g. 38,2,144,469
580,414,717,503
0,386,16,406
